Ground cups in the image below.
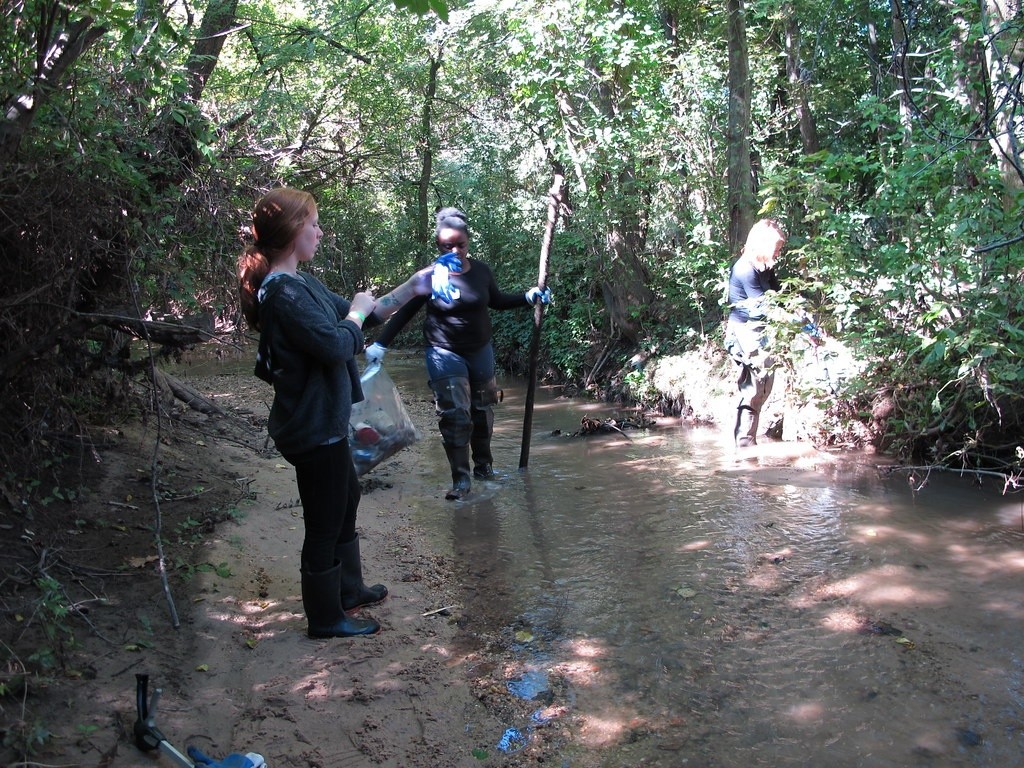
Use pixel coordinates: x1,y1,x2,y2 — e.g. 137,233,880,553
371,409,394,435
355,423,377,443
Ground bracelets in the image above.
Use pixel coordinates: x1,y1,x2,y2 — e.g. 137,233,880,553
351,311,365,322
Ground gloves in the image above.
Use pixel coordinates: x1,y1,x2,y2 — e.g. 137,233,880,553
364,341,387,368
428,275,456,304
524,284,553,308
430,252,463,274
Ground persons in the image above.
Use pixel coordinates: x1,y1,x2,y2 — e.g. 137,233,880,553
365,207,550,499
234,189,447,640
723,220,813,449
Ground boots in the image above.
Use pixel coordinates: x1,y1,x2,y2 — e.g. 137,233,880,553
470,406,495,480
299,558,382,640
334,532,389,612
441,440,472,500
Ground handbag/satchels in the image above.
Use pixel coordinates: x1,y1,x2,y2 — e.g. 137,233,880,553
347,361,422,477
